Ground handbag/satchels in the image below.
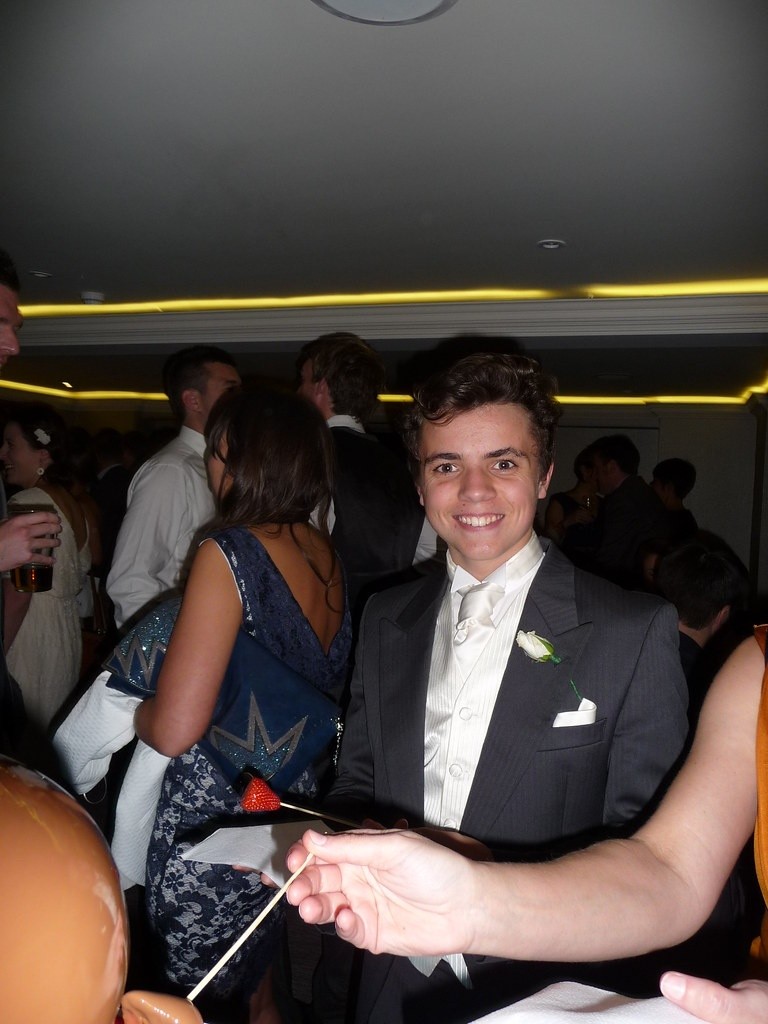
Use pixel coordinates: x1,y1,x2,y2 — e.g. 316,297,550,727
100,589,343,796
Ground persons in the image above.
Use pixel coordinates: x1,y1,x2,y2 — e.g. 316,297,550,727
105,346,241,638
545,433,699,592
100,381,353,1024
295,331,395,639
653,528,739,735
232,351,691,1024
54,425,151,585
0,245,63,755
285,620,768,1024
0,400,92,755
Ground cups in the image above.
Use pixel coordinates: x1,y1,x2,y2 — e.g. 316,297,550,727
581,496,593,509
6,503,58,592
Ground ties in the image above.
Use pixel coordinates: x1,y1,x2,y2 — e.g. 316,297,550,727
451,580,506,689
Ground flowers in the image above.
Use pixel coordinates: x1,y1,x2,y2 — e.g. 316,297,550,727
517,629,583,703
